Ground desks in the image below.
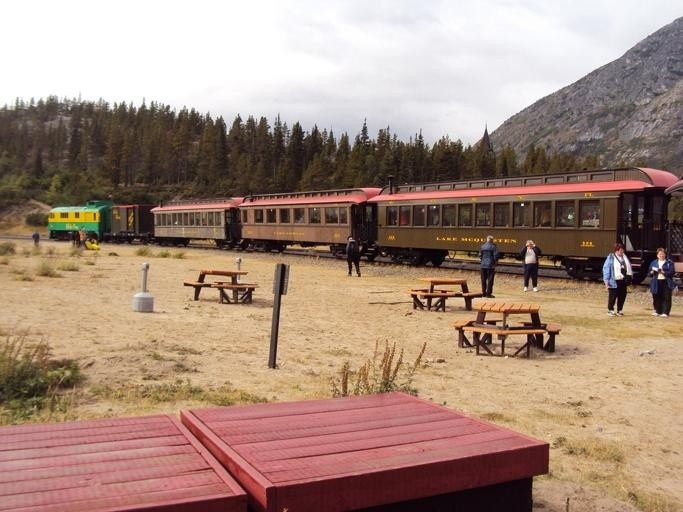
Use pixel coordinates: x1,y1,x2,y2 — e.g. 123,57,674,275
194,267,248,304
470,301,545,350
418,277,471,311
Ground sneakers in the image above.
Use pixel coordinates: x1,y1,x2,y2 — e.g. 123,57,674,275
533,287,538,292
483,293,495,298
652,313,658,316
616,311,623,316
524,287,527,291
660,314,667,318
607,310,615,316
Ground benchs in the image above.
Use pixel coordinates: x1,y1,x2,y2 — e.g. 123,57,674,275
209,284,258,305
182,280,213,301
516,320,561,352
409,291,456,313
454,317,503,347
462,290,483,310
410,287,453,310
213,280,258,301
465,324,545,359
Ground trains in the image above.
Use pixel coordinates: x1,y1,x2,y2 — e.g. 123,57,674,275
45,160,683,300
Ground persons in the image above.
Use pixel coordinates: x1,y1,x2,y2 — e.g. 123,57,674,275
32,230,39,246
521,239,541,292
602,243,633,317
479,235,498,298
647,247,675,318
345,236,361,277
71,228,98,249
